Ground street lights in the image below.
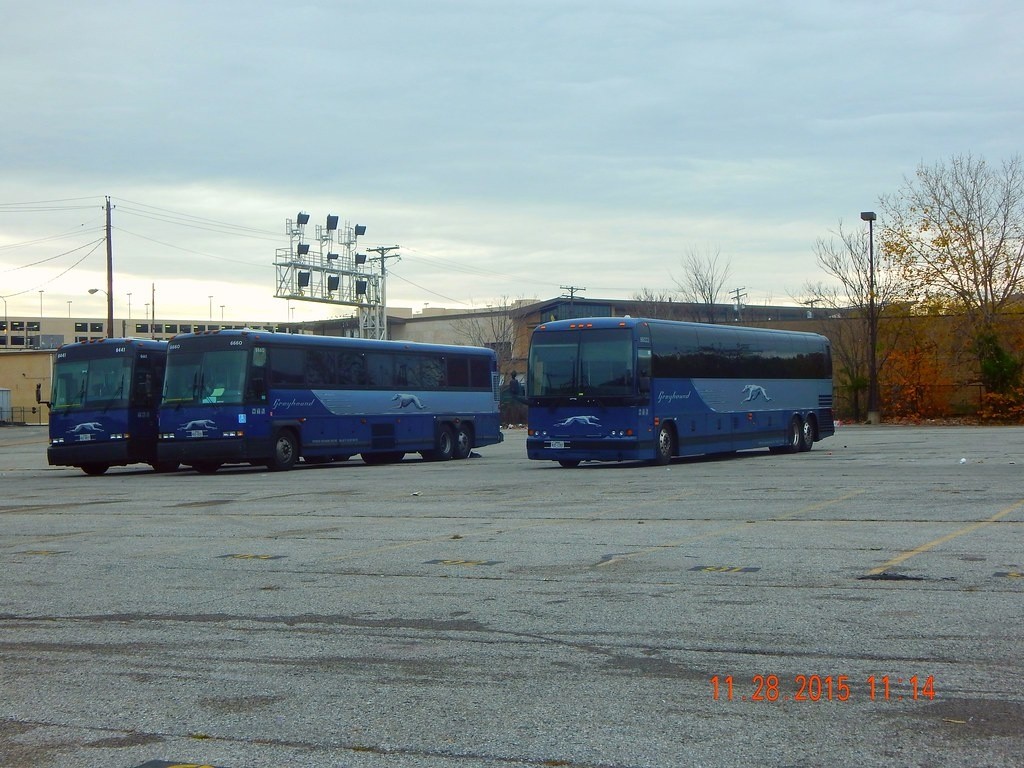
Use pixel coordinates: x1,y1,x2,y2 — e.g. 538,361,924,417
145,304,150,319
424,302,429,308
221,306,225,321
89,289,113,338
67,301,72,318
860,212,882,424
39,290,44,316
127,292,132,318
208,296,214,321
290,308,295,322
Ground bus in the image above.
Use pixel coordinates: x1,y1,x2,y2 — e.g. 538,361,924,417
156,329,505,474
36,337,169,475
526,315,836,469
